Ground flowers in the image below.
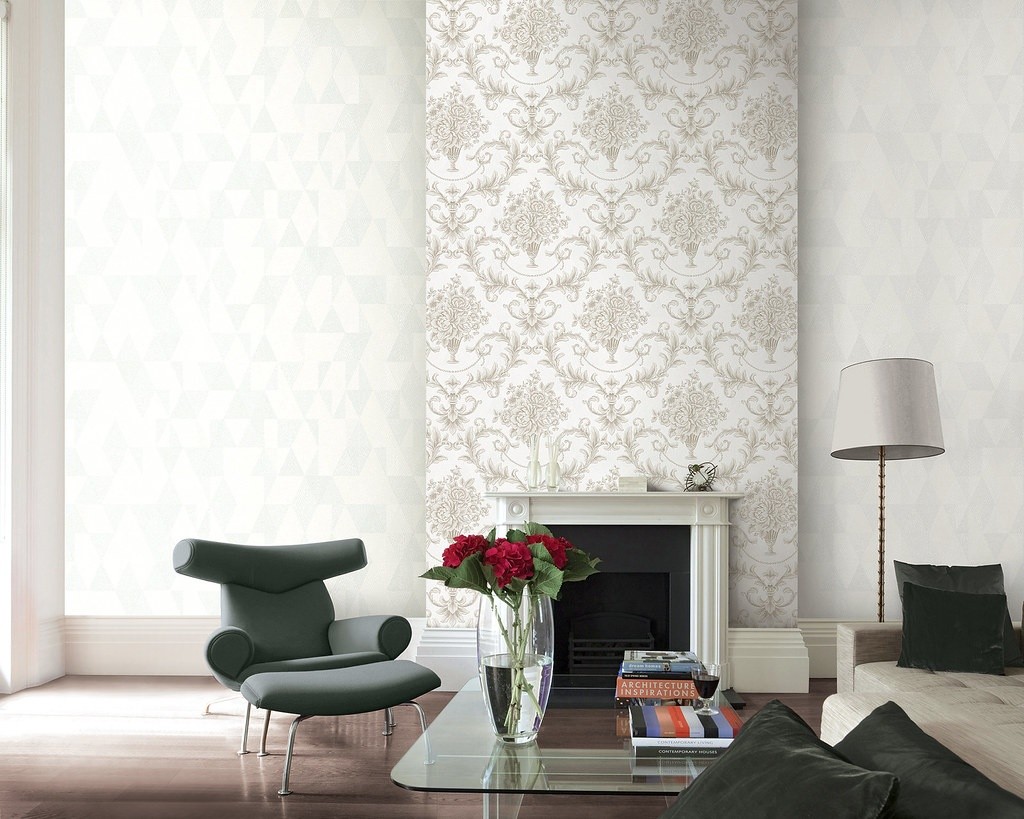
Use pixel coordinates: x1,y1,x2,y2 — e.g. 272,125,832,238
419,519,601,734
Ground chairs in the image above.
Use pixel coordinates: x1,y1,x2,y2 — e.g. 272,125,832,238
173,538,441,796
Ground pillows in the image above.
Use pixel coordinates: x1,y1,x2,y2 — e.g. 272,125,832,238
659,700,897,819
892,560,1019,666
896,582,1006,676
833,700,1024,819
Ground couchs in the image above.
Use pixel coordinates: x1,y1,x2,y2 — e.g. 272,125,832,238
820,623,1024,800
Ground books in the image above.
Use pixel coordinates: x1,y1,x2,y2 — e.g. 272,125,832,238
629,705,743,758
629,759,713,783
615,708,630,749
614,650,708,708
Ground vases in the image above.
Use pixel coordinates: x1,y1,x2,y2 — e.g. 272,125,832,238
476,590,557,744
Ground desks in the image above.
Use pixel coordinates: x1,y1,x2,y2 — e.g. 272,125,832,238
388,678,743,819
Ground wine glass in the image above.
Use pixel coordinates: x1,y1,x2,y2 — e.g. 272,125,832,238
690,664,722,716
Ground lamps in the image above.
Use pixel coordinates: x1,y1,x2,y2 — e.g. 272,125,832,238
830,357,944,622
